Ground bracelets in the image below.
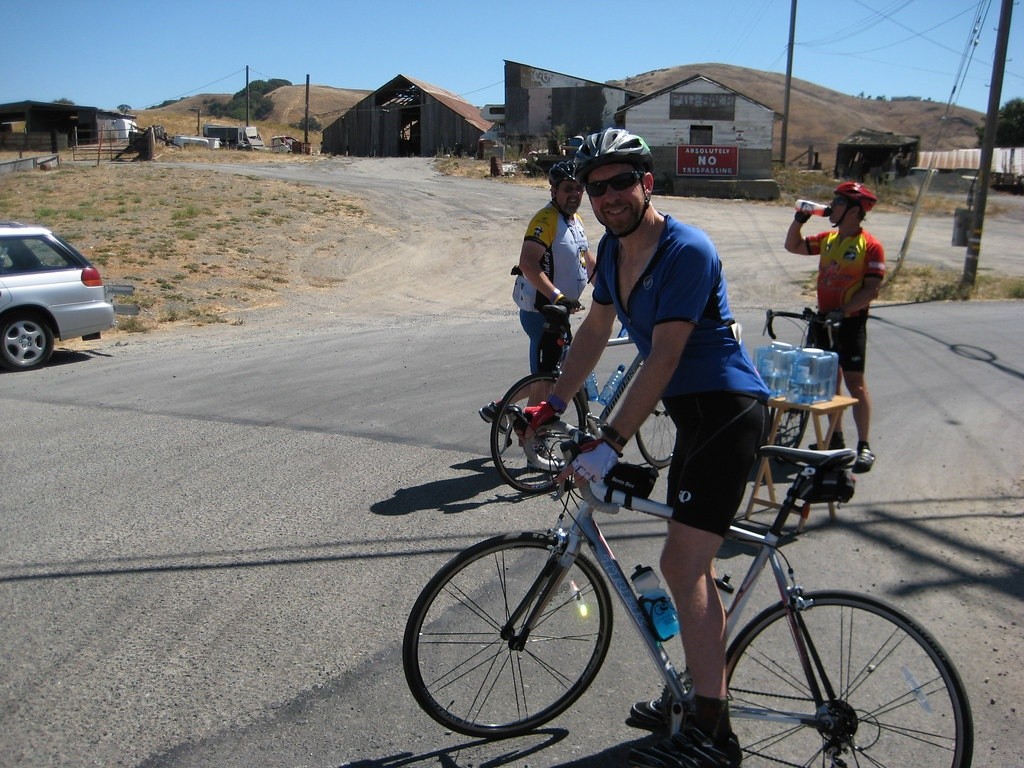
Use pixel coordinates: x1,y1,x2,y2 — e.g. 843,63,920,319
602,424,628,447
546,394,567,414
550,287,565,304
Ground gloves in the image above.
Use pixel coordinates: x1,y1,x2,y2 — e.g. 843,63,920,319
795,202,813,223
825,309,844,321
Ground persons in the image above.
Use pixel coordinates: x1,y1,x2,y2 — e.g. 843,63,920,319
784,182,886,472
517,130,772,768
512,162,599,471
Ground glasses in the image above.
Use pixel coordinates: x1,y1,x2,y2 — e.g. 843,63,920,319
565,185,584,193
586,171,641,197
833,196,846,205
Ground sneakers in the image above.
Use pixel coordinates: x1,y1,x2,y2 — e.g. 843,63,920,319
627,724,743,768
528,444,565,471
479,402,508,434
630,696,671,724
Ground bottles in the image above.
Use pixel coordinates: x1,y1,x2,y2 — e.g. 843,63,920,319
753,340,839,404
714,574,735,616
795,199,833,217
584,370,599,402
630,565,680,640
598,364,625,407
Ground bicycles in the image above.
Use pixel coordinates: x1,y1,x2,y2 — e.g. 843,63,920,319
402,403,975,768
490,304,676,495
762,306,840,465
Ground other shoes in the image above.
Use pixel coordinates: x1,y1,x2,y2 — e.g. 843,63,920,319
808,440,845,450
856,449,875,471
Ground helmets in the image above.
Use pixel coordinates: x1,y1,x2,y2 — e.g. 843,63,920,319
548,161,575,181
833,180,879,212
575,127,656,183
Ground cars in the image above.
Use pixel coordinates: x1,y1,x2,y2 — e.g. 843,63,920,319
0,221,140,372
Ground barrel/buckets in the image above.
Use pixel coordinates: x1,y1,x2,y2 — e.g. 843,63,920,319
547,139,561,155
292,141,302,155
491,157,504,176
302,143,312,155
